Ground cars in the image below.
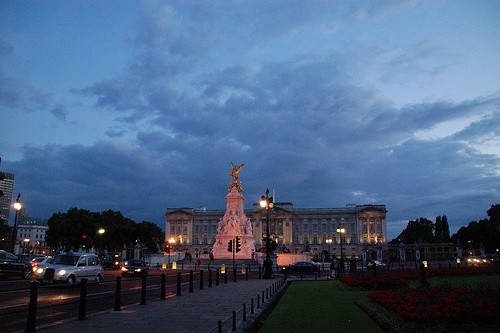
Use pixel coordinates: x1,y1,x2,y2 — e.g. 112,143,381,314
281,262,318,274
121,260,149,277
367,260,387,269
35,251,104,286
0,250,51,281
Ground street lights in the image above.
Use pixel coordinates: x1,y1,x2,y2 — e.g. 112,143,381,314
259,188,275,278
98,228,105,257
337,224,346,276
11,200,22,253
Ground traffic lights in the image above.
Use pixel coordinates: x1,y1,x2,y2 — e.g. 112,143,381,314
236,236,241,253
227,239,233,252
